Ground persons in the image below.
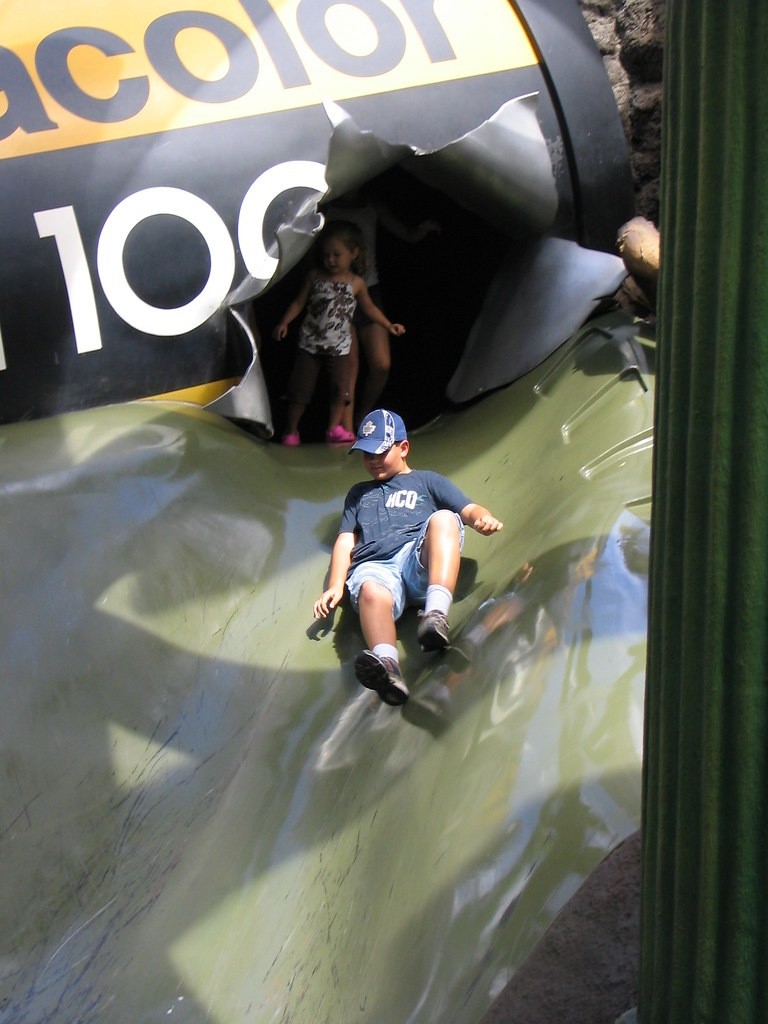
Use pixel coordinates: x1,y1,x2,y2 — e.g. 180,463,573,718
269,220,405,446
318,178,445,432
312,408,504,707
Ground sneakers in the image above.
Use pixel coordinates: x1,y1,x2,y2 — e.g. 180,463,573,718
354,651,407,706
438,639,476,672
402,696,452,736
417,610,450,652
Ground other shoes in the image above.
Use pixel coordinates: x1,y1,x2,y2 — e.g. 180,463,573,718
284,433,300,446
326,425,353,442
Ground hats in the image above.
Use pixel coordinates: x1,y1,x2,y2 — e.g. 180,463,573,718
348,409,406,457
